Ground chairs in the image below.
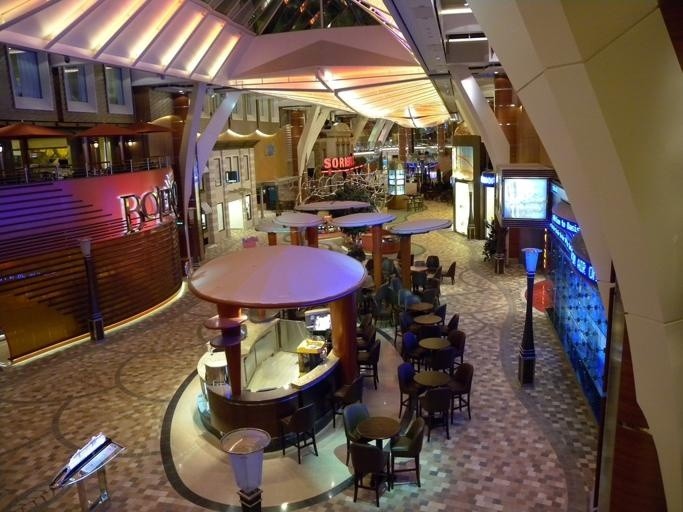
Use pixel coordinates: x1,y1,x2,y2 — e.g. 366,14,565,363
342,403,370,465
278,401,318,465
403,181,453,213
350,442,391,508
331,374,364,428
390,415,425,489
353,254,474,443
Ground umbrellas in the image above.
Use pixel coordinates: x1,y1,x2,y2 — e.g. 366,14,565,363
128,119,175,158
0,119,73,182
73,122,138,169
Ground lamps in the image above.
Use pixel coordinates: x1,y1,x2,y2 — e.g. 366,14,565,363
126,136,137,147
206,88,216,99
63,56,70,63
159,74,165,80
90,138,99,149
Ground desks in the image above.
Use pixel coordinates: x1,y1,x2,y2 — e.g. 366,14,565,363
355,416,401,487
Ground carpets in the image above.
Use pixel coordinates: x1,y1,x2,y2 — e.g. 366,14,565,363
525,278,550,313
318,230,343,239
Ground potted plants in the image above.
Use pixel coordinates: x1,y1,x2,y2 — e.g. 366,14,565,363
322,182,373,248
482,220,497,269
